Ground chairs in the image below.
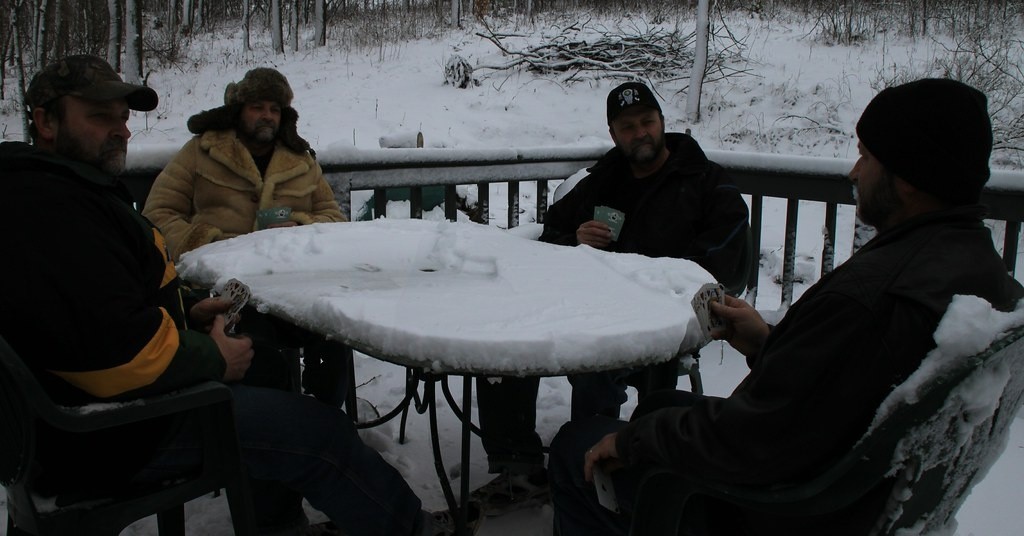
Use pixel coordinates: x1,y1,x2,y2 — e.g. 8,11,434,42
582,323,1024,536
611,185,753,425
285,340,358,424
0,331,242,536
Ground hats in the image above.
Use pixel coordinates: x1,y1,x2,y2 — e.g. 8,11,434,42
607,82,662,126
187,67,309,155
24,55,158,120
857,79,993,206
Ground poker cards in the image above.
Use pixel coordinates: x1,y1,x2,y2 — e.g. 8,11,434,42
593,205,625,242
691,283,728,340
591,463,621,515
219,278,251,327
259,205,293,231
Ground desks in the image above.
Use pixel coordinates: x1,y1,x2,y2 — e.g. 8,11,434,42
174,217,726,536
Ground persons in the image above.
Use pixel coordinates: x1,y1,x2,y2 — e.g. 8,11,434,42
548,77,992,536
468,82,752,523
1,56,425,536
141,66,357,427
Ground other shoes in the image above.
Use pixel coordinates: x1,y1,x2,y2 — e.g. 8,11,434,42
429,500,484,536
472,467,544,515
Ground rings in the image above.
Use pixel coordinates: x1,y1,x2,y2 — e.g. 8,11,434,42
589,449,593,453
